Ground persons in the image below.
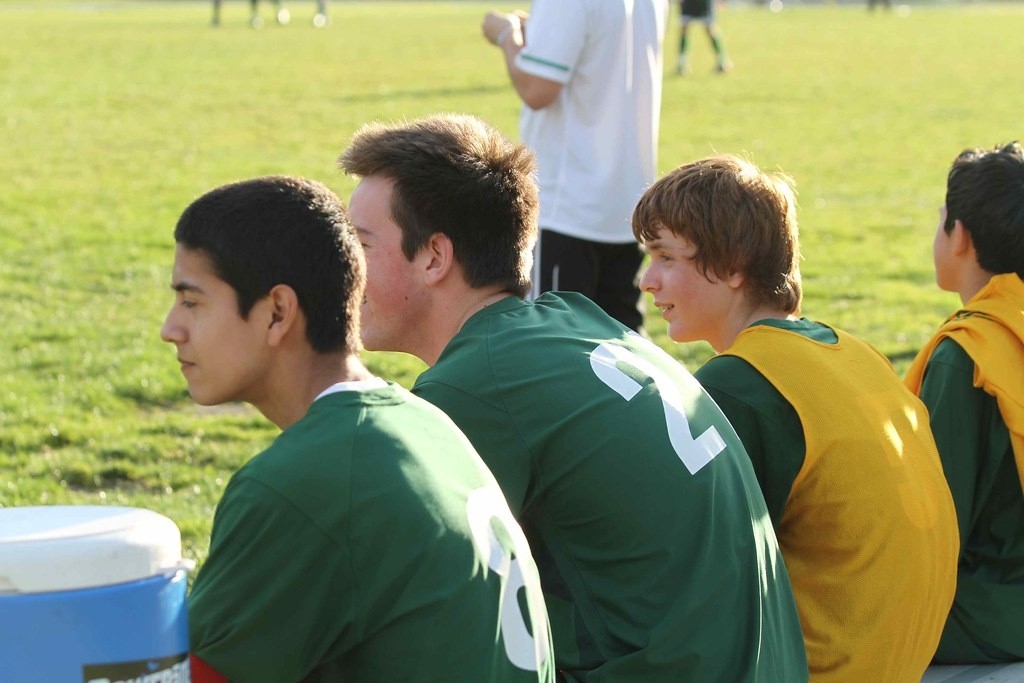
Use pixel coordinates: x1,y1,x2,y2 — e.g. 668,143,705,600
676,0,727,73
868,0,891,13
271,0,327,26
336,112,809,683
900,139,1024,666
631,153,961,683
482,0,669,342
161,175,556,683
211,0,261,28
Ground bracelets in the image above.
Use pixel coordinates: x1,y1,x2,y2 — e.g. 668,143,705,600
496,25,521,46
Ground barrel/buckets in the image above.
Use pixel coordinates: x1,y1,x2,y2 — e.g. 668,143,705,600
0,505,193,683
